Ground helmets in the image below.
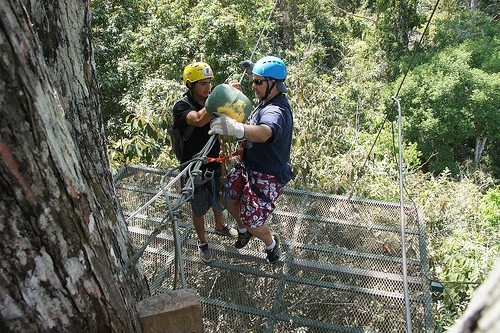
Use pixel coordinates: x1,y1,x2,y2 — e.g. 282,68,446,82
182,62,215,86
250,56,287,81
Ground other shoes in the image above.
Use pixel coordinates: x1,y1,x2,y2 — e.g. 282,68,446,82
197,238,212,262
215,223,239,237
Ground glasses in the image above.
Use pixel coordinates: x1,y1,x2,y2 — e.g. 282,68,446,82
253,79,272,85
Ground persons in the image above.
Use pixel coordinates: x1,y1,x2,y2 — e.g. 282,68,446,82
207,55,293,265
169,62,238,263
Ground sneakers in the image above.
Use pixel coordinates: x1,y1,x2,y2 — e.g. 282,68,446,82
234,227,256,249
264,232,281,266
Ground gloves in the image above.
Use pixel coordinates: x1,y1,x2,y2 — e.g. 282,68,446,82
207,112,244,140
239,60,254,75
